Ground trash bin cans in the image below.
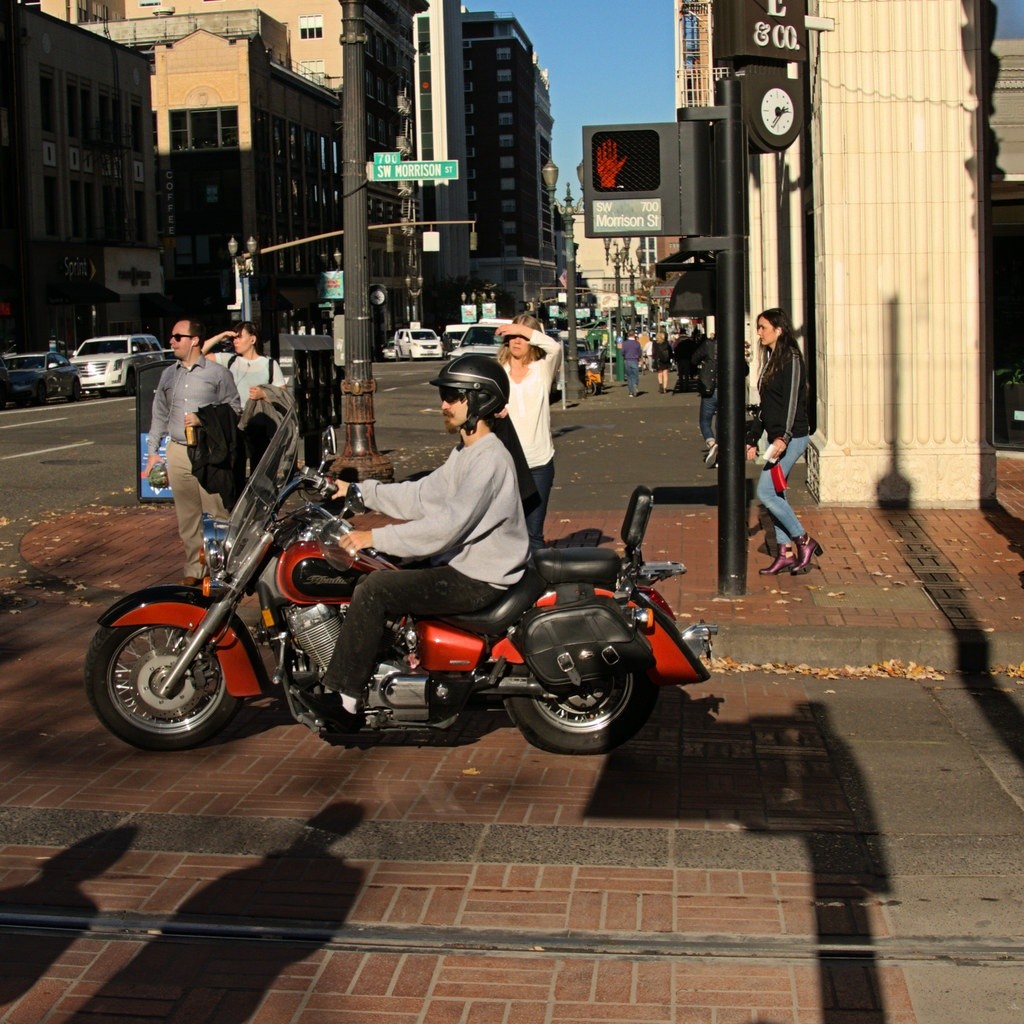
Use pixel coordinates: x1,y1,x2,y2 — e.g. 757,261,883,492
49,335,68,356
136,359,179,503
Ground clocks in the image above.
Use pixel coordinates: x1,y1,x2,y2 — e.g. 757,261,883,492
747,57,803,152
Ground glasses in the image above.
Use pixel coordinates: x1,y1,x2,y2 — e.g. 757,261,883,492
438,385,468,404
167,332,195,342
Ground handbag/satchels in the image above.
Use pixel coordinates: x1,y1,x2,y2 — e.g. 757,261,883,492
696,340,716,399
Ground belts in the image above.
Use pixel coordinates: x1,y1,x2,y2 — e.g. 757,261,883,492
171,439,188,447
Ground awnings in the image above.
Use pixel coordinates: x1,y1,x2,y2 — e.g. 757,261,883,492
668,271,716,317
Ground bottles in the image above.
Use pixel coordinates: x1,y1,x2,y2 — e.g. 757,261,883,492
237,398,256,431
184,411,197,445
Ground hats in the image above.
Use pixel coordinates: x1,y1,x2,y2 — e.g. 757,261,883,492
680,329,686,334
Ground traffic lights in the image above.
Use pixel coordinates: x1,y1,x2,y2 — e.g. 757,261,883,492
577,119,710,240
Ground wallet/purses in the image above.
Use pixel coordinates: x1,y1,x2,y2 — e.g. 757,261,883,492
771,458,787,493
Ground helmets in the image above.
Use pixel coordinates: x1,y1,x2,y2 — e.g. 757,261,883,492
430,354,510,419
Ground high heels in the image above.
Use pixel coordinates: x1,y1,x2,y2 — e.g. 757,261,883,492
759,544,799,576
791,532,823,573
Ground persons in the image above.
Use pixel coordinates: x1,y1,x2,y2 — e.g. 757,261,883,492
495,314,563,541
287,353,531,731
621,328,749,467
746,308,822,575
144,318,287,586
1,334,24,369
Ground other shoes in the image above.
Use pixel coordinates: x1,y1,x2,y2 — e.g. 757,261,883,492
628,391,633,397
290,684,362,738
633,384,638,396
658,387,663,393
705,443,718,466
663,389,666,393
176,576,202,587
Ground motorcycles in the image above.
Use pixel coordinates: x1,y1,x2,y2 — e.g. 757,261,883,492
82,408,722,754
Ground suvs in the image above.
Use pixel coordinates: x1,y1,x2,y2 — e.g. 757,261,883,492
68,333,165,398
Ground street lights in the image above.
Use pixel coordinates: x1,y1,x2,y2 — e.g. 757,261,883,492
320,246,344,315
619,247,645,330
542,157,584,399
228,234,257,321
461,291,476,324
603,236,633,340
403,273,424,321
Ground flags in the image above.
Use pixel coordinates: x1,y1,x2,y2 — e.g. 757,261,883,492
559,270,567,287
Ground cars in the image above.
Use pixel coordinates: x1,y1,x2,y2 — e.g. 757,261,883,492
380,319,516,363
0,350,82,411
546,321,681,397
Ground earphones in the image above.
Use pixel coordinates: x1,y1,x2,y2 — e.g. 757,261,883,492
191,341,195,346
252,339,254,342
